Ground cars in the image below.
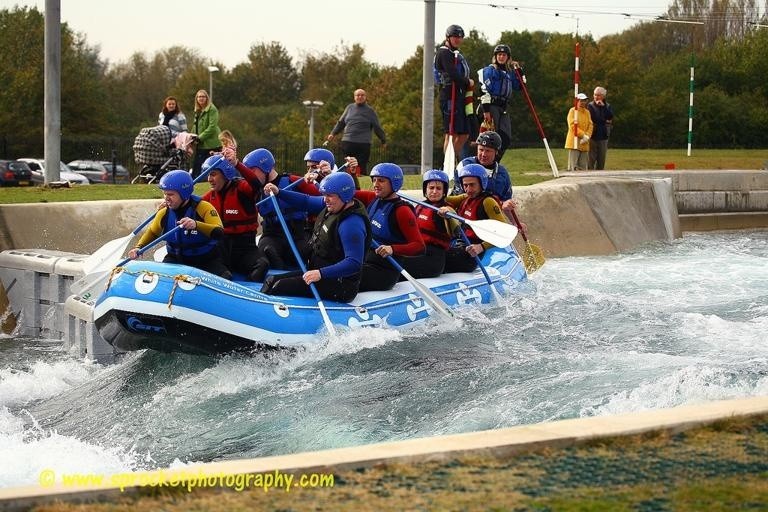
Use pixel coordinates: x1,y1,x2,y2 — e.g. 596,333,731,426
0,161,30,187
16,158,89,184
67,159,130,184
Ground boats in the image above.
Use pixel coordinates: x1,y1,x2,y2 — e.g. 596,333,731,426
93,242,531,356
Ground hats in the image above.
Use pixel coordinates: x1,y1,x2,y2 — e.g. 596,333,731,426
577,93,589,100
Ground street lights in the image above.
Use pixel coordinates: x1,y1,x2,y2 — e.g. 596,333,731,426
302,99,324,152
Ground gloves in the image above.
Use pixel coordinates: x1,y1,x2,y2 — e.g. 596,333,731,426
580,138,586,145
583,134,589,144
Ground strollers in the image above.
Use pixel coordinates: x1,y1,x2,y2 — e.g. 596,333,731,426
130,124,196,184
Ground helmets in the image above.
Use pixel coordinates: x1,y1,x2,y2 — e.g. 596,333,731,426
445,24,465,38
475,131,503,151
494,44,511,56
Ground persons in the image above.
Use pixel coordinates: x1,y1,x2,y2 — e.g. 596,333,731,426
475,42,527,169
435,22,479,165
564,90,594,169
325,86,387,177
156,96,189,140
181,88,227,180
127,130,528,303
586,87,614,171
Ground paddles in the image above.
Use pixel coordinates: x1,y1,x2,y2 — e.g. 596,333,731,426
513,64,560,177
310,175,456,320
69,224,181,302
443,50,459,181
154,173,308,263
267,189,346,353
82,156,226,276
511,208,546,275
395,193,518,248
453,221,506,312
